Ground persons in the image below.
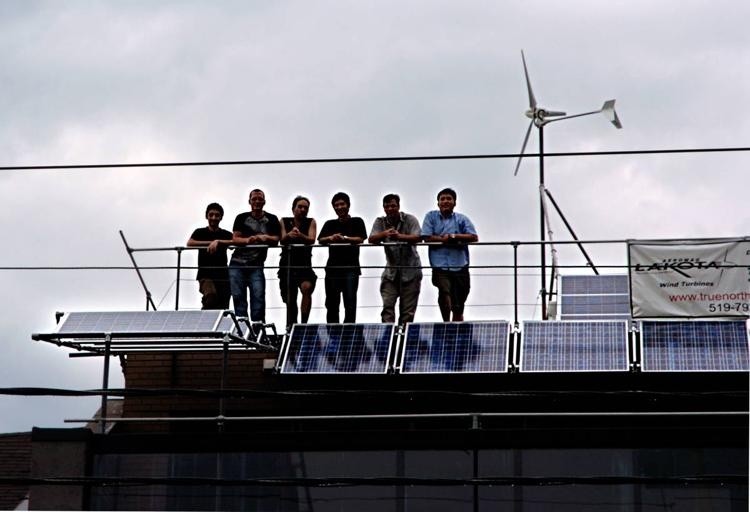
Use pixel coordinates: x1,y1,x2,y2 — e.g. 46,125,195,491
368,193,425,333
420,187,478,322
187,203,233,317
229,189,281,343
277,196,317,333
317,192,367,323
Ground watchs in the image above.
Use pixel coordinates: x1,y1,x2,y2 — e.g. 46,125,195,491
448,234,457,241
